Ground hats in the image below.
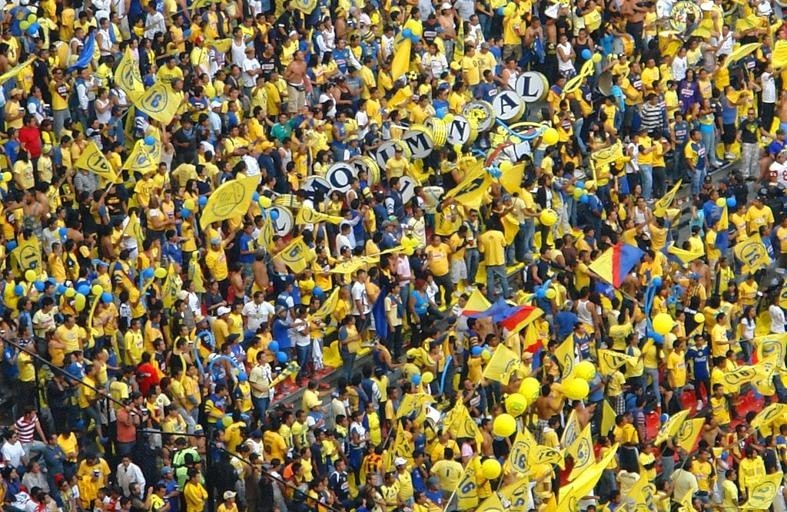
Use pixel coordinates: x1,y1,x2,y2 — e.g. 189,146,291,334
395,457,407,468
223,490,237,501
216,306,230,317
381,219,396,228
441,2,452,11
227,333,241,344
261,141,274,151
10,87,22,97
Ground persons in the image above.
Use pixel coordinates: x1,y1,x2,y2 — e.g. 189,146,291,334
1,1,787,512
